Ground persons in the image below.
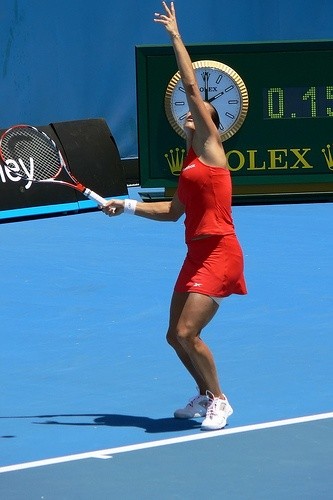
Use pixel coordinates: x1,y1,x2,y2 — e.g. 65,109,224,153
97,0,247,431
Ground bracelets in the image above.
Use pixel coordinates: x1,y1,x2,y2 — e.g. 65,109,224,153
123,198,137,216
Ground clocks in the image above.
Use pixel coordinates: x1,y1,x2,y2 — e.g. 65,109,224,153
164,60,248,141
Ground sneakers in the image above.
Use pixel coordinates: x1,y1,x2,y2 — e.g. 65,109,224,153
201,389,233,430
174,395,213,418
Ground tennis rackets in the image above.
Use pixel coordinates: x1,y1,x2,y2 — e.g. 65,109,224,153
0,125,117,215
